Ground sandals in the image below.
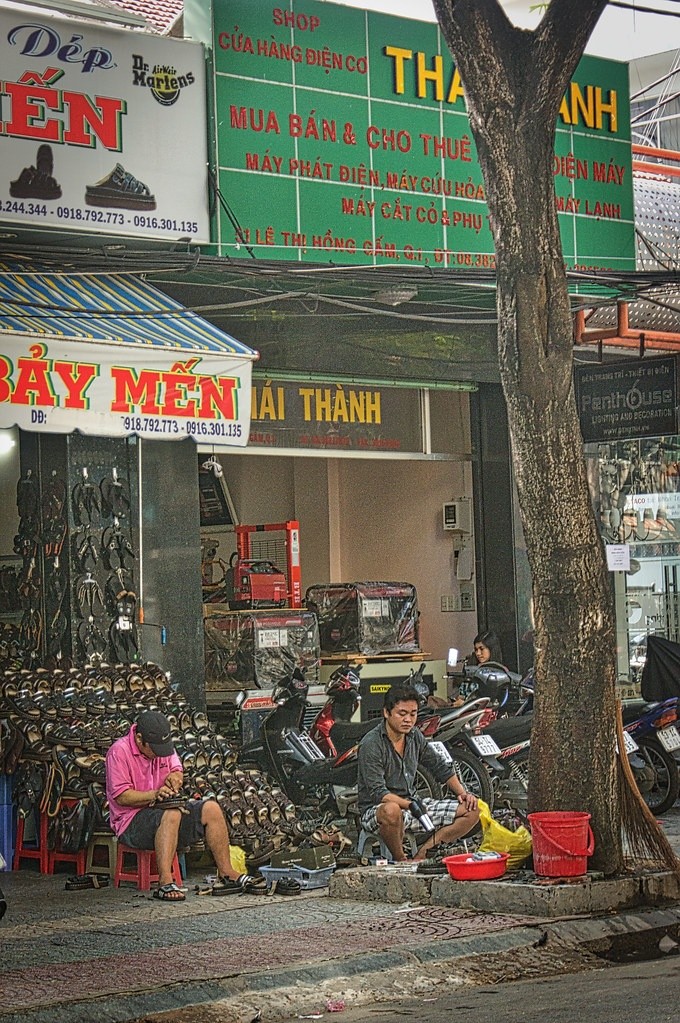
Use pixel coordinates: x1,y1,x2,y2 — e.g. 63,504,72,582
249,813,361,868
212,873,301,897
0,661,296,852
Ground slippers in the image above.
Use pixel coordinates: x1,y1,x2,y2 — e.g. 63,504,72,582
153,882,185,901
0,475,138,664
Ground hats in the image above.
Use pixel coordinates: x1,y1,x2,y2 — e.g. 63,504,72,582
136,711,174,757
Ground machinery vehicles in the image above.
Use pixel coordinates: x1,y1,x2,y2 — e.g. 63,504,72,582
204,520,432,691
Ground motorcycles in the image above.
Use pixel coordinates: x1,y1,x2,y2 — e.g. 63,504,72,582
236,659,680,840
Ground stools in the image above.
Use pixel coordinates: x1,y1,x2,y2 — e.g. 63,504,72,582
0,768,418,891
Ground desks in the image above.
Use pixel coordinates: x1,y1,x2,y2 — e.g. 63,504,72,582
323,652,449,721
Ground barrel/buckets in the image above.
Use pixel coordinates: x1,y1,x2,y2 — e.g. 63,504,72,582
528,811,596,877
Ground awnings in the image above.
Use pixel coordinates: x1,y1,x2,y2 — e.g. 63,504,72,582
0,257,259,448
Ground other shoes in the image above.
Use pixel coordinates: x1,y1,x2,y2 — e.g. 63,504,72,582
65,875,108,889
155,789,189,809
418,856,448,875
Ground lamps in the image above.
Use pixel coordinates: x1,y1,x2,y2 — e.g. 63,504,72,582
374,283,419,308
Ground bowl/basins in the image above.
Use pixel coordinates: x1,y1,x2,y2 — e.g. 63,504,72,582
441,852,511,880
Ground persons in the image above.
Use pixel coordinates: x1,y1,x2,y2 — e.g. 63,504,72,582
457,629,502,698
356,684,481,861
104,711,265,901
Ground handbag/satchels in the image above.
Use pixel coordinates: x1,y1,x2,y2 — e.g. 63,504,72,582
476,798,533,869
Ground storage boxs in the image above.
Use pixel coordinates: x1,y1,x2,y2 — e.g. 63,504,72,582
271,846,336,871
259,864,336,890
